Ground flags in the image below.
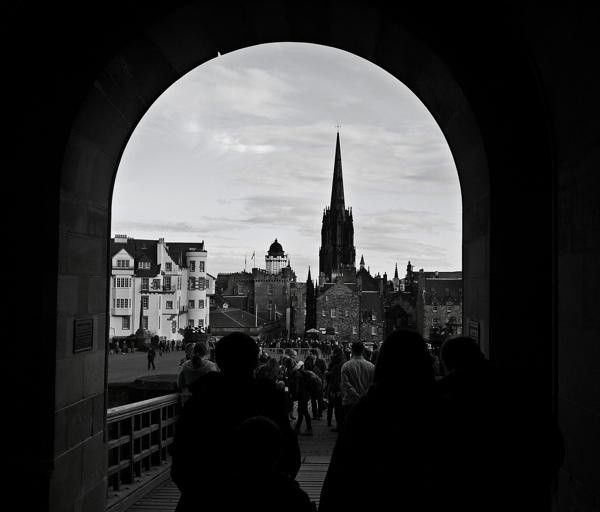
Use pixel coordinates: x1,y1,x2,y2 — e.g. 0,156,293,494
251,251,256,259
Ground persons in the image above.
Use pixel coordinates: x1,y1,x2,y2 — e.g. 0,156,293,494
147,323,379,430
122,340,129,353
434,331,567,510
314,325,439,511
114,341,122,355
169,331,317,511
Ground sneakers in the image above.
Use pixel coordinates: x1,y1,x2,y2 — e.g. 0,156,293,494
295,428,313,436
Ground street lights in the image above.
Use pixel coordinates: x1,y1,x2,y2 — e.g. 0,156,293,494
357,294,362,340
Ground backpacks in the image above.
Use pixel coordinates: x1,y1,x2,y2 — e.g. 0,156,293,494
299,370,322,396
